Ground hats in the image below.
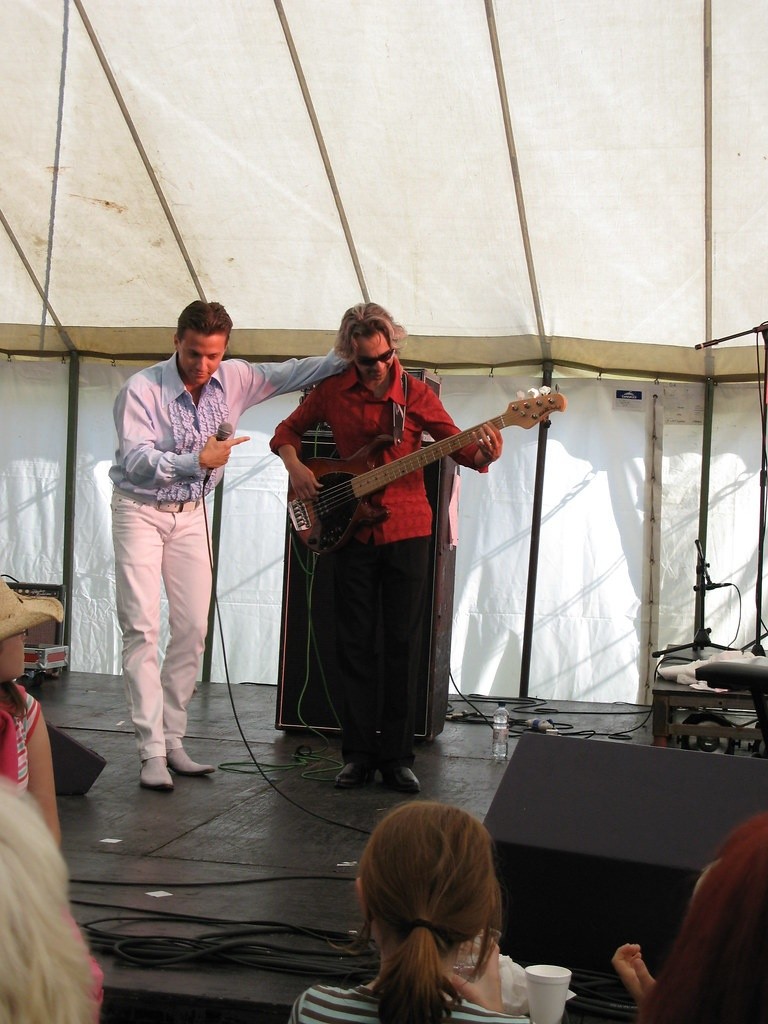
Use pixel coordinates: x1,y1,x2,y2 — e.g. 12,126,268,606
0,578,64,639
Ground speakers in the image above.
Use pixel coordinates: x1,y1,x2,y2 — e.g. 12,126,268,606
47,721,106,796
483,733,768,978
5,581,66,644
274,436,462,741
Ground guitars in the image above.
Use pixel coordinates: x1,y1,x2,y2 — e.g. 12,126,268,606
287,384,567,554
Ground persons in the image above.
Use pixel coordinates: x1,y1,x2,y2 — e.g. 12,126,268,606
611,810,768,1024
269,303,503,792
290,801,534,1023
109,299,349,790
0,778,105,1024
0,577,66,847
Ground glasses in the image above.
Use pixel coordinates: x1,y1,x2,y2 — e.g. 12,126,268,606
354,348,395,365
0,629,29,644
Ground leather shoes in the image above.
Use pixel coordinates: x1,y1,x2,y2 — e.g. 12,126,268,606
167,746,215,776
139,748,174,790
381,758,420,793
335,760,375,793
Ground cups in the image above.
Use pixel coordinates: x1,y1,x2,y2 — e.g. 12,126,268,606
452,925,502,984
523,963,573,1023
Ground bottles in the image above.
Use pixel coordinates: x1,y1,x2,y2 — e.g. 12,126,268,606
490,700,510,760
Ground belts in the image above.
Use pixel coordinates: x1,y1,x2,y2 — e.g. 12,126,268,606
114,484,204,513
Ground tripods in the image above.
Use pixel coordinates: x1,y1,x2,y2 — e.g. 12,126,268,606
651,539,738,658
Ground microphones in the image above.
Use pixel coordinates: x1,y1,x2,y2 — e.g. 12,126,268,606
203,422,233,486
693,583,732,592
